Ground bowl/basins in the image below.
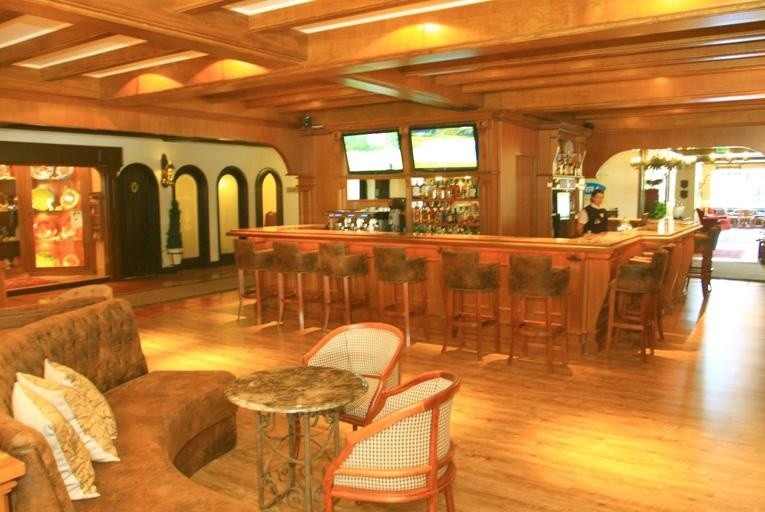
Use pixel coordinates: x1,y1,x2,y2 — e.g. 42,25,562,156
560,179,576,189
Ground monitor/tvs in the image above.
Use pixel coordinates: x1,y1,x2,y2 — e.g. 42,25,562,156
409,123,478,171
342,128,403,174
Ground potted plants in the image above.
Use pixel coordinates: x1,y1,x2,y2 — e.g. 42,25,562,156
167,192,183,265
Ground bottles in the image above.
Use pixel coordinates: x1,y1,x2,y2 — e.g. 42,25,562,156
559,154,582,176
415,177,480,199
413,203,479,235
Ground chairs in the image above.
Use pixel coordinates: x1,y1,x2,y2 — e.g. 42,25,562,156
439,249,501,359
288,321,405,461
605,209,720,361
272,240,319,331
373,246,429,348
320,243,368,331
507,252,570,372
232,239,272,324
323,372,456,512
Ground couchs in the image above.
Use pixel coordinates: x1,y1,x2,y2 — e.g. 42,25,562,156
0,297,251,512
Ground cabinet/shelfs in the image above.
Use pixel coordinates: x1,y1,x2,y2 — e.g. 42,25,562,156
411,176,479,227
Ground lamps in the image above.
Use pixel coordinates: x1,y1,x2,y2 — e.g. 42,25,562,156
160,153,176,188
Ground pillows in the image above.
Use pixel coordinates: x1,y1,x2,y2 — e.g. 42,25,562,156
13,359,120,499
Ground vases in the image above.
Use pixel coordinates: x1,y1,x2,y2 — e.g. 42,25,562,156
672,201,686,218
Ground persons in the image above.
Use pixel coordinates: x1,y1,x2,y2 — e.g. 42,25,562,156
576,188,609,237
